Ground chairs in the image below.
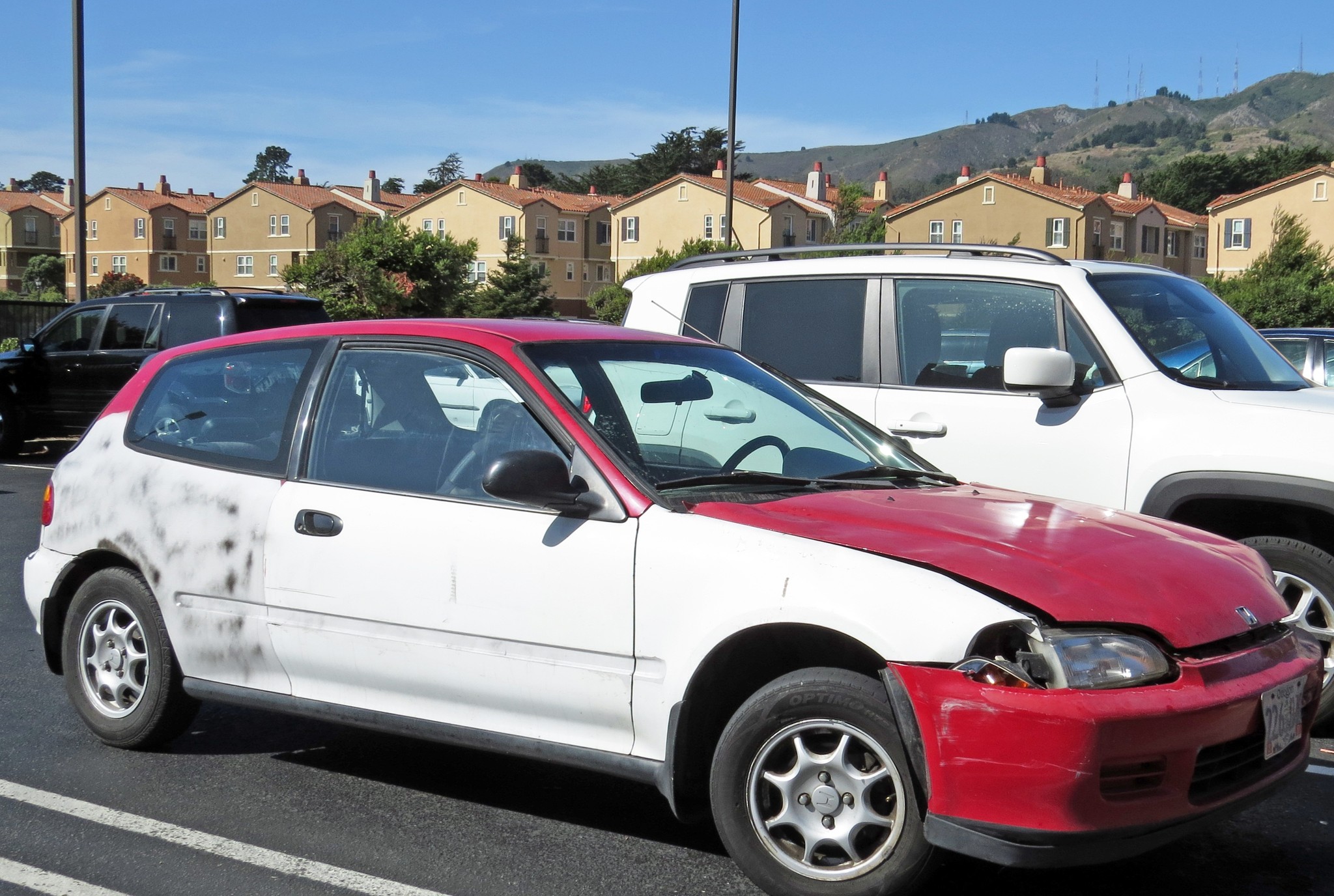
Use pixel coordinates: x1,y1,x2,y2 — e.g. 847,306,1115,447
970,304,1053,390
903,306,944,390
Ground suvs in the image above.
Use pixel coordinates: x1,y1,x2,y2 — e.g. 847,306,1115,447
585,237,1334,736
0,286,332,457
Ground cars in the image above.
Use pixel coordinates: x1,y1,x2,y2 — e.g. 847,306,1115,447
23,315,1323,896
421,357,585,432
937,326,1334,394
